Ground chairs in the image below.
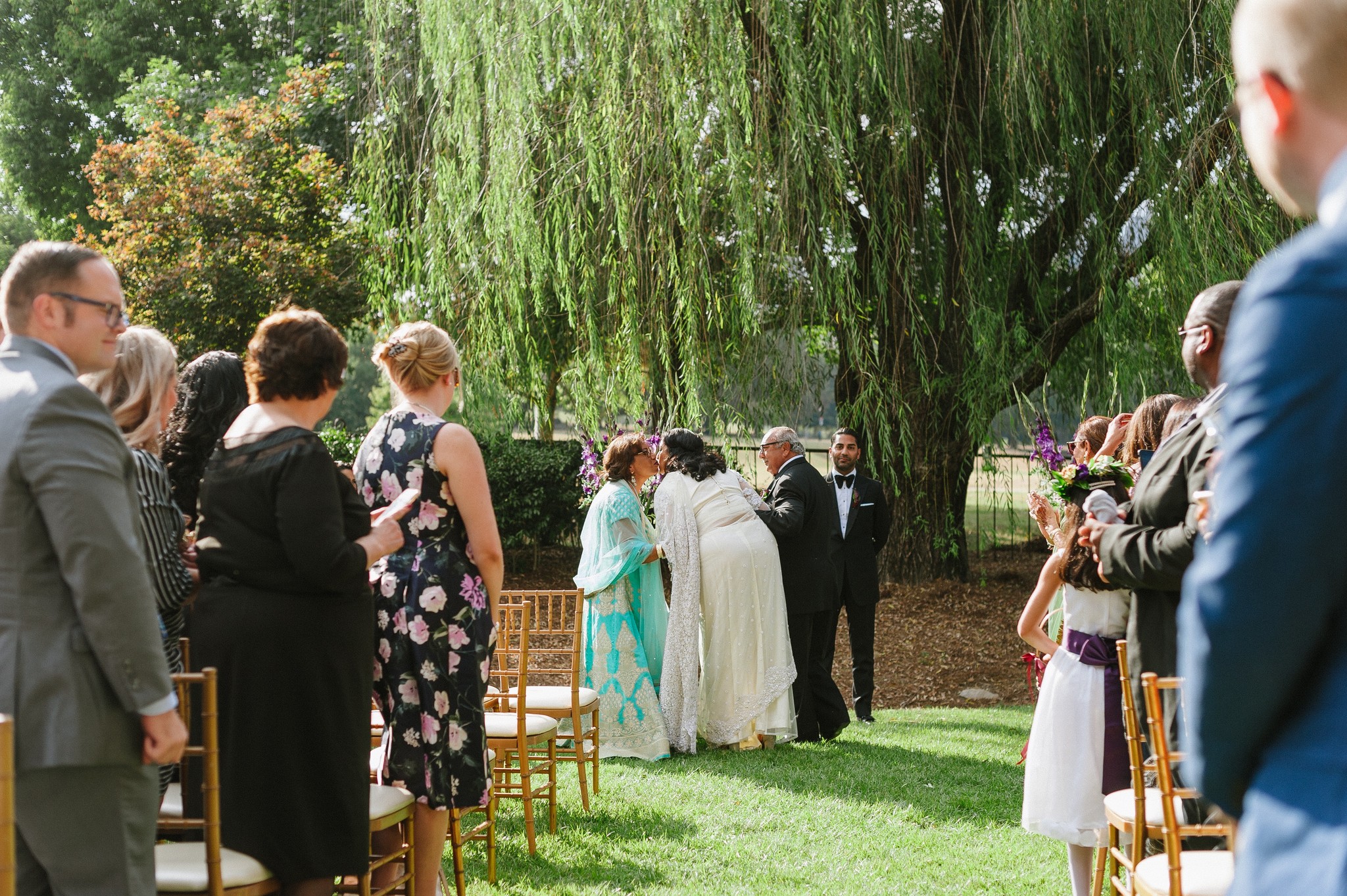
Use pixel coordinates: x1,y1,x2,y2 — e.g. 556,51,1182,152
1,584,605,894
1093,641,1245,896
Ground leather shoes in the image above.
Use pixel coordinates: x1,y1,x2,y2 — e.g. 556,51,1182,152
825,712,851,741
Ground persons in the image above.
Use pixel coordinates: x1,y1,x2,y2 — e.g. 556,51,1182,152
753,426,850,744
159,348,248,526
0,238,184,896
195,308,413,893
1015,459,1145,895
1160,395,1202,440
354,319,505,896
554,433,670,765
1090,392,1179,467
1176,0,1346,893
821,427,890,726
1079,278,1243,850
1027,414,1129,645
78,323,197,817
652,429,798,754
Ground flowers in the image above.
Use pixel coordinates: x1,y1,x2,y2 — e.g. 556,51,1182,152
1034,415,1080,503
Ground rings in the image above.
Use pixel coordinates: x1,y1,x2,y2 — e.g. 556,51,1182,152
1031,509,1034,514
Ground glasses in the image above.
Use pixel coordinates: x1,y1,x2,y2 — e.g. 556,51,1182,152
48,292,129,328
759,440,787,454
1178,326,1206,340
632,448,651,458
1067,441,1081,456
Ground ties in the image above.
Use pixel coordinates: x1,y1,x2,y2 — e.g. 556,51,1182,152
834,474,855,489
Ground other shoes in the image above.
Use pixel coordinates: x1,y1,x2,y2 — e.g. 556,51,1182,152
858,716,875,723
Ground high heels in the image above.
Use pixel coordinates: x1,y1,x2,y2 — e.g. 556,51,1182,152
706,742,740,753
763,735,776,750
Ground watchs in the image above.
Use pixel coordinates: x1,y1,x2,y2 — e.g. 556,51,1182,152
1050,528,1061,539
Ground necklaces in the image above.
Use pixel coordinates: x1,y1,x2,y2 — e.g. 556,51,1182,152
627,480,646,512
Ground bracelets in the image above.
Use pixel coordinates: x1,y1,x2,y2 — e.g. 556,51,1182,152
1046,538,1054,550
656,543,664,559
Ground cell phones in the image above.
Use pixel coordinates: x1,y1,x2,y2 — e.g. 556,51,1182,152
369,487,421,527
1137,448,1155,470
